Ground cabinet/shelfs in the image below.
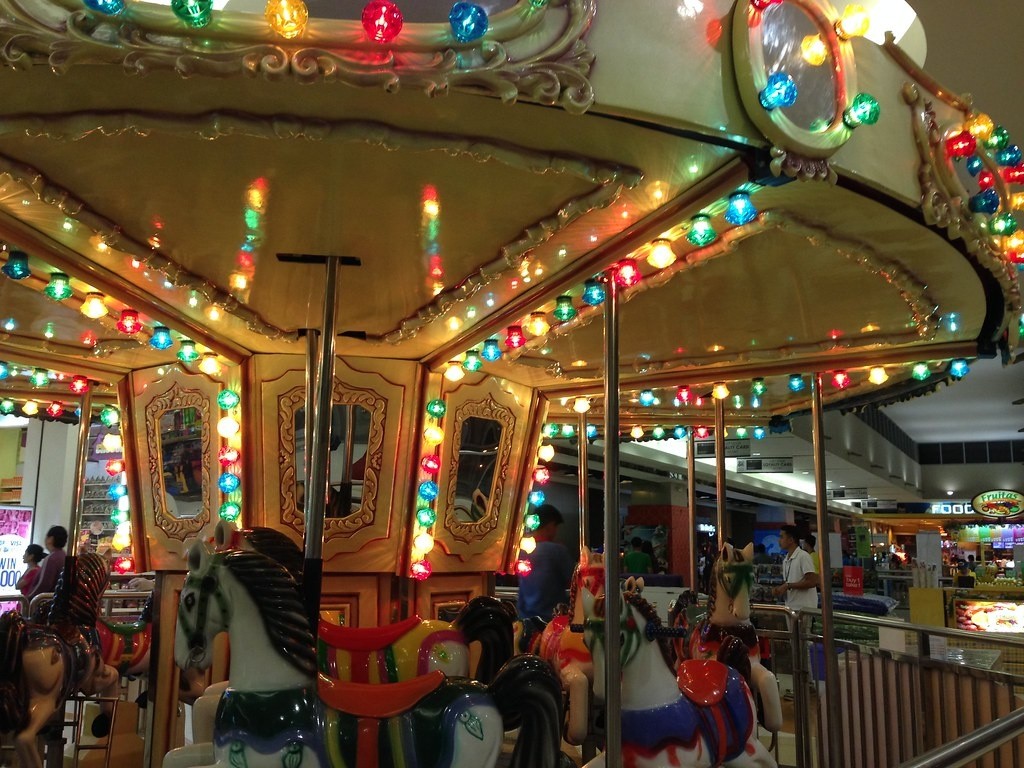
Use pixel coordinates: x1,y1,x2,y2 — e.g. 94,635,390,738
77,483,134,558
0,486,22,502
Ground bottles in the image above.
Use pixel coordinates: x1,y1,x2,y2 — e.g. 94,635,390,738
974,561,1022,587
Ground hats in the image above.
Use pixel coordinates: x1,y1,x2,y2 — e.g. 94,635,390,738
958,559,970,570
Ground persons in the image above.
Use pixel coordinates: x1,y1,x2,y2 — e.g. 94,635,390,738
516,504,575,623
802,534,821,593
754,544,769,565
771,525,820,698
951,558,976,587
696,550,706,592
623,536,654,574
15,544,43,613
967,554,977,572
26,524,68,601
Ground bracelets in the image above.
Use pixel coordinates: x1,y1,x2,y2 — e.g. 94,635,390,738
787,583,792,590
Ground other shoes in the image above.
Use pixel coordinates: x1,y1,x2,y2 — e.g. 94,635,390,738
786,685,817,696
784,694,795,700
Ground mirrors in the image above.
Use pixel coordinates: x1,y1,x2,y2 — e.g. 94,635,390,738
277,376,389,542
443,392,518,544
144,381,211,542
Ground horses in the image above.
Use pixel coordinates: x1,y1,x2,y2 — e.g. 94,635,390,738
192,520,514,744
667,543,783,732
0,547,119,768
162,538,563,768
582,576,778,768
513,547,607,745
34,589,154,709
